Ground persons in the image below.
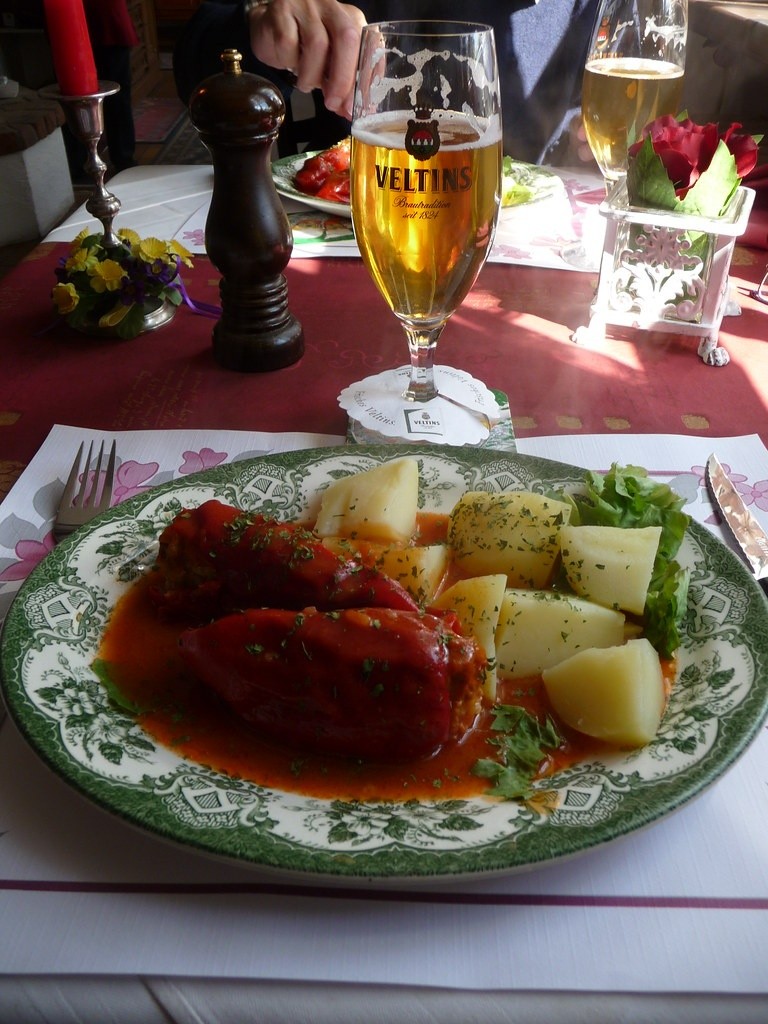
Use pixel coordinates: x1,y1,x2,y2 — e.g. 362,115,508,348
172,1,639,166
60,0,141,181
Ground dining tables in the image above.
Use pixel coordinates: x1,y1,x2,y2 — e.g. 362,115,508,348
1,162,768,1023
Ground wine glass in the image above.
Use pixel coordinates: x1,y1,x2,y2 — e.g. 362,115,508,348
581,3,692,213
340,21,513,455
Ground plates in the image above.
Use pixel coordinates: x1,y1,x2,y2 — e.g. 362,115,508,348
9,444,768,872
266,142,558,218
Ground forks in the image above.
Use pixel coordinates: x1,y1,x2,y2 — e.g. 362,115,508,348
50,442,120,547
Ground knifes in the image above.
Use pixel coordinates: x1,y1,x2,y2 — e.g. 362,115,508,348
703,448,768,604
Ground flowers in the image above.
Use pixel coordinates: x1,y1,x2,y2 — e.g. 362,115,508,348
51,226,195,340
624,108,765,311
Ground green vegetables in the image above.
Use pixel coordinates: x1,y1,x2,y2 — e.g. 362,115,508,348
470,463,690,807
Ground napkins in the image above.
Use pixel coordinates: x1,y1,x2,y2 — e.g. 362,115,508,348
1,422,768,995
164,162,650,273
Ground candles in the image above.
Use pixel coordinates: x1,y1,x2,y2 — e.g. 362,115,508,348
43,0,99,96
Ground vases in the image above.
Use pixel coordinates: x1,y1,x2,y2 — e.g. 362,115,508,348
570,175,757,367
